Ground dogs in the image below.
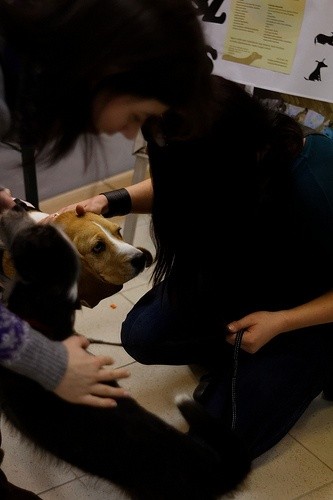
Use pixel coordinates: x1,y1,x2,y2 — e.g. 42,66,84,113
0,212,146,308
0,206,252,500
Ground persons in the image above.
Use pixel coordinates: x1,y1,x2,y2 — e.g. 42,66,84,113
55,75,333,459
0,0,211,500
